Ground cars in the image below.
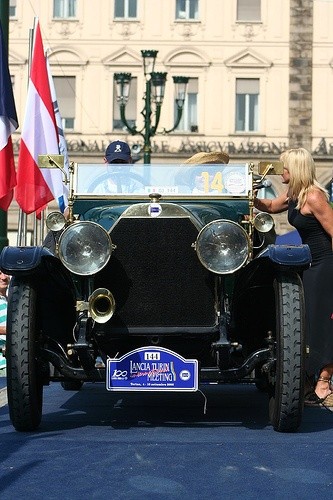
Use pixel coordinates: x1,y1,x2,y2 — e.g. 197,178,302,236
1,153,314,435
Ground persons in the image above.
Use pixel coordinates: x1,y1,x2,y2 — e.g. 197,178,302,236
177,147,333,409
0,273,11,378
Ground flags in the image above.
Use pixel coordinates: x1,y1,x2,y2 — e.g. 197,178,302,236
0,0,70,222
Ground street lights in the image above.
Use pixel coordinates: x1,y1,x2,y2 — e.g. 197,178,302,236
113,49,190,164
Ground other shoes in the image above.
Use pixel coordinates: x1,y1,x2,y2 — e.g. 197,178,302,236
301,376,333,404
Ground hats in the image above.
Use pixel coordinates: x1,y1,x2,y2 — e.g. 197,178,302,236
183,151,230,174
105,141,132,163
